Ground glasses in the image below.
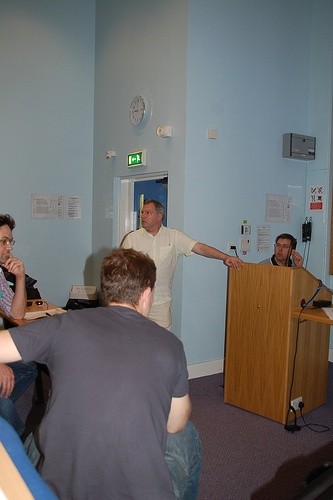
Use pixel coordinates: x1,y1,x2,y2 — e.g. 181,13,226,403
26,300,48,310
0,238,15,245
274,244,290,249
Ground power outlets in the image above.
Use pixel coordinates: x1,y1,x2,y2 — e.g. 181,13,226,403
227,242,237,252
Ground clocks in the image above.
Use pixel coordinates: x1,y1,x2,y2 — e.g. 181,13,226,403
128,96,146,127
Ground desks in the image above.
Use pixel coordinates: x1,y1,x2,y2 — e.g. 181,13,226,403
293,308,333,325
0,299,57,326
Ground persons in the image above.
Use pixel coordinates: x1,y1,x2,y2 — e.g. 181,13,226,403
0,248,203,500
0,213,41,436
258,233,303,267
120,199,245,331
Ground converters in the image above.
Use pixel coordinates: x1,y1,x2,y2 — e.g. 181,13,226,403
312,299,331,308
